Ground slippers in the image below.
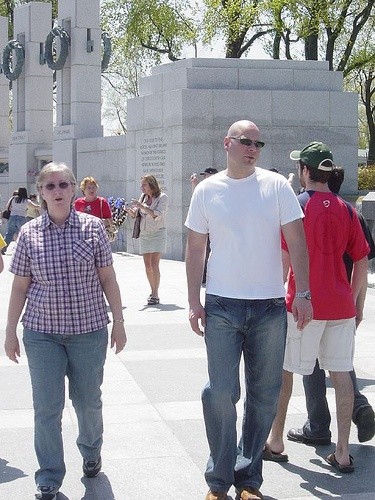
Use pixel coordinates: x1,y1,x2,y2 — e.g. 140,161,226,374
262,442,289,461
325,450,354,473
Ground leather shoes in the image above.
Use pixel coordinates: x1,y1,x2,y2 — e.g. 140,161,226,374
287,428,332,445
357,405,375,442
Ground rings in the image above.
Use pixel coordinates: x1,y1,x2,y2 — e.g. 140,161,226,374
308,316,311,320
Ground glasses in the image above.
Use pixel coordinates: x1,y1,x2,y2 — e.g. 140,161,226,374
47,182,69,190
229,136,265,148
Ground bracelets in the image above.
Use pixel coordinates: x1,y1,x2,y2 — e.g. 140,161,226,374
114,319,125,323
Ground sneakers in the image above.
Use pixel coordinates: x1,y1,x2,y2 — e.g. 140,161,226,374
205,488,227,500
235,488,264,500
83,455,102,477
202,284,206,288
35,483,59,500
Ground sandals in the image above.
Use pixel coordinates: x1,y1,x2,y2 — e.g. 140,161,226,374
148,295,159,305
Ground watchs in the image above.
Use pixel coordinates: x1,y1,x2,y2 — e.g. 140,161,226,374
295,290,311,300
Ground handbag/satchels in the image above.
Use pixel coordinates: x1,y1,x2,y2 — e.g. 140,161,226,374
132,194,147,239
101,197,117,242
3,197,15,219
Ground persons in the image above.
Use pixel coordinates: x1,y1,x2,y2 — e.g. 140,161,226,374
0,233,7,275
190,167,218,288
261,142,370,475
13,191,18,242
73,177,113,228
268,167,295,187
1,187,41,255
288,164,375,446
185,120,315,500
126,175,169,305
25,194,40,223
5,161,128,500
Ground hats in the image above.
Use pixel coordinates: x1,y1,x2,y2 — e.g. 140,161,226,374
200,167,218,175
290,141,334,171
